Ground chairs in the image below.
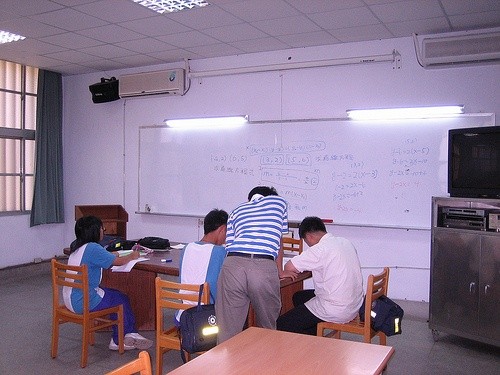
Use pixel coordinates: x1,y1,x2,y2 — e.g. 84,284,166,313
281,237,304,255
316,265,390,372
103,350,152,375
49,258,125,369
154,276,210,375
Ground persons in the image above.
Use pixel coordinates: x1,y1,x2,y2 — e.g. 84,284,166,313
62,215,154,351
174,209,229,340
276,215,367,337
214,185,299,349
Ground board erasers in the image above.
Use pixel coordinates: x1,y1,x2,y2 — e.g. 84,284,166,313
321,218,334,224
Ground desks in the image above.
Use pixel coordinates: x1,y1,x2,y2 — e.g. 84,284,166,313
165,325,394,375
64,240,313,333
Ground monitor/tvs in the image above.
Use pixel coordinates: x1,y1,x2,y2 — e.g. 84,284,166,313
447,125,500,199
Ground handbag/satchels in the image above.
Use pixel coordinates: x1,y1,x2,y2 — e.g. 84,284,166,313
359,290,404,337
105,240,139,252
138,237,170,249
180,284,246,354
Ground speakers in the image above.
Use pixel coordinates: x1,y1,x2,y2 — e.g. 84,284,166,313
89,79,121,104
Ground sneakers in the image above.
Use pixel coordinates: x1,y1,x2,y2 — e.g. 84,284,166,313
124,333,153,349
109,337,135,350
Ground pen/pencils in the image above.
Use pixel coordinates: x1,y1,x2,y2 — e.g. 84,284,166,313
135,244,140,250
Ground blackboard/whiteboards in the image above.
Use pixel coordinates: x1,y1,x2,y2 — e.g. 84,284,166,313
135,112,496,230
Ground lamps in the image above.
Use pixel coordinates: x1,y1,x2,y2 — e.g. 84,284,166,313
345,103,465,120
163,114,249,129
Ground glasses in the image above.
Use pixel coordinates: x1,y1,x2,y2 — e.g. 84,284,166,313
100,227,106,231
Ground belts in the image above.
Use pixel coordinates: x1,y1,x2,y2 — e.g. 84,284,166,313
227,252,274,261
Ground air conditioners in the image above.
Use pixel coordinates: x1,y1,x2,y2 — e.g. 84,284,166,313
420,31,500,68
118,68,186,99
